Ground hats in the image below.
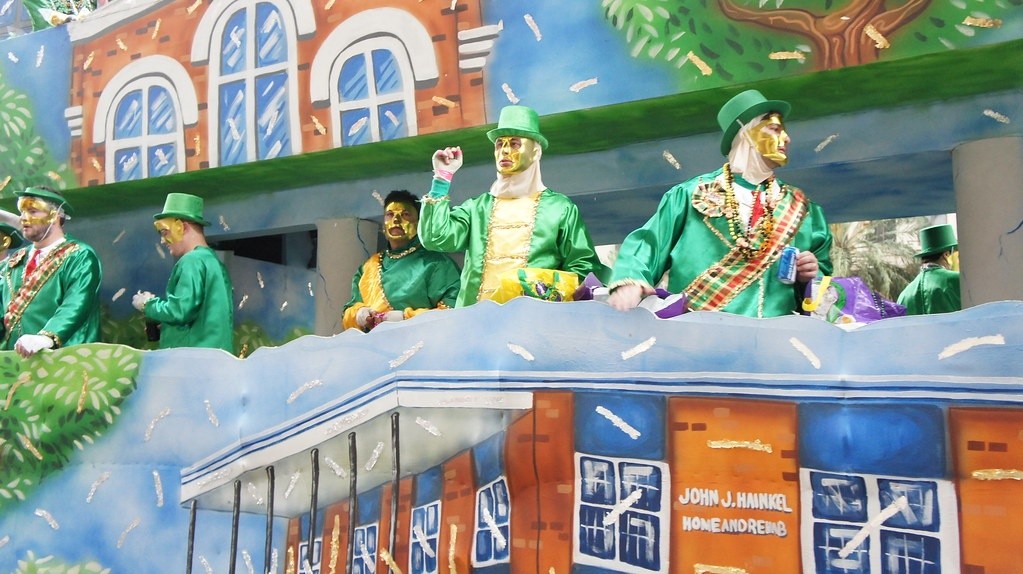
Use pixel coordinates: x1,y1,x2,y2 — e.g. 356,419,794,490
716,88,792,158
153,192,211,227
912,226,958,258
12,187,73,217
0,207,26,248
486,105,548,151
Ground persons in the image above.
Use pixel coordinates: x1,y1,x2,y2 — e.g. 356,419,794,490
341,189,462,333
417,105,602,309
21,0,98,31
607,89,834,317
896,224,960,315
0,184,103,358
132,193,233,354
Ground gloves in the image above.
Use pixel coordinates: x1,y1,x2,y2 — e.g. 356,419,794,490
131,290,155,311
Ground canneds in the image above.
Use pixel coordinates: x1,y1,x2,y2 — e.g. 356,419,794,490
777,246,800,284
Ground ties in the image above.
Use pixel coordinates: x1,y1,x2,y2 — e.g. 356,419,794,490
25,250,40,278
750,190,764,237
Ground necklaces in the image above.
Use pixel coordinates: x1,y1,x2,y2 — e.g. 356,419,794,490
386,244,419,259
723,162,774,260
